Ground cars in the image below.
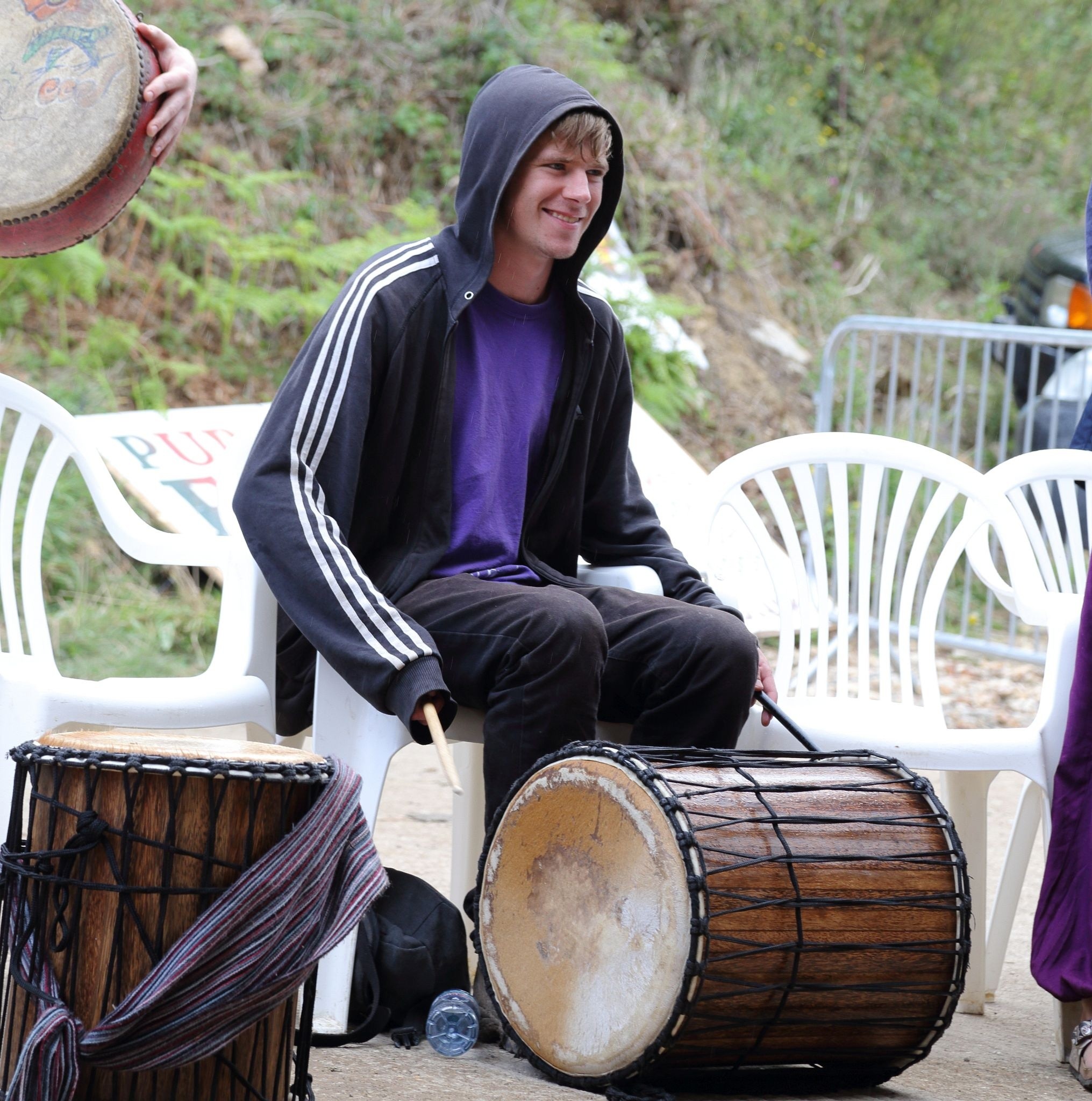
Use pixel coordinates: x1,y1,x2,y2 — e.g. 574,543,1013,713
989,228,1091,453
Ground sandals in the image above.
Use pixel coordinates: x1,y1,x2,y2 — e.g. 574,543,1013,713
1069,1019,1092,1086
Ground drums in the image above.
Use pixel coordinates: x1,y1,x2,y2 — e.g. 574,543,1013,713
0,1,158,262
5,725,333,1100
471,743,977,1090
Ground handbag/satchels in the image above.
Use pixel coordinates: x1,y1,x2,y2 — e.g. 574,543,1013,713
291,864,473,1051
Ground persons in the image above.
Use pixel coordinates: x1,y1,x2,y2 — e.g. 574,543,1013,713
137,22,198,169
1029,181,1092,1094
233,66,777,1058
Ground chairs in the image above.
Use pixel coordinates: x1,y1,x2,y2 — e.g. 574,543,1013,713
0,373,278,847
690,431,1052,1015
311,398,831,1034
963,449,1092,1002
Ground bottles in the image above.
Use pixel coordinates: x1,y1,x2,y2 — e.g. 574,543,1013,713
426,989,480,1056
